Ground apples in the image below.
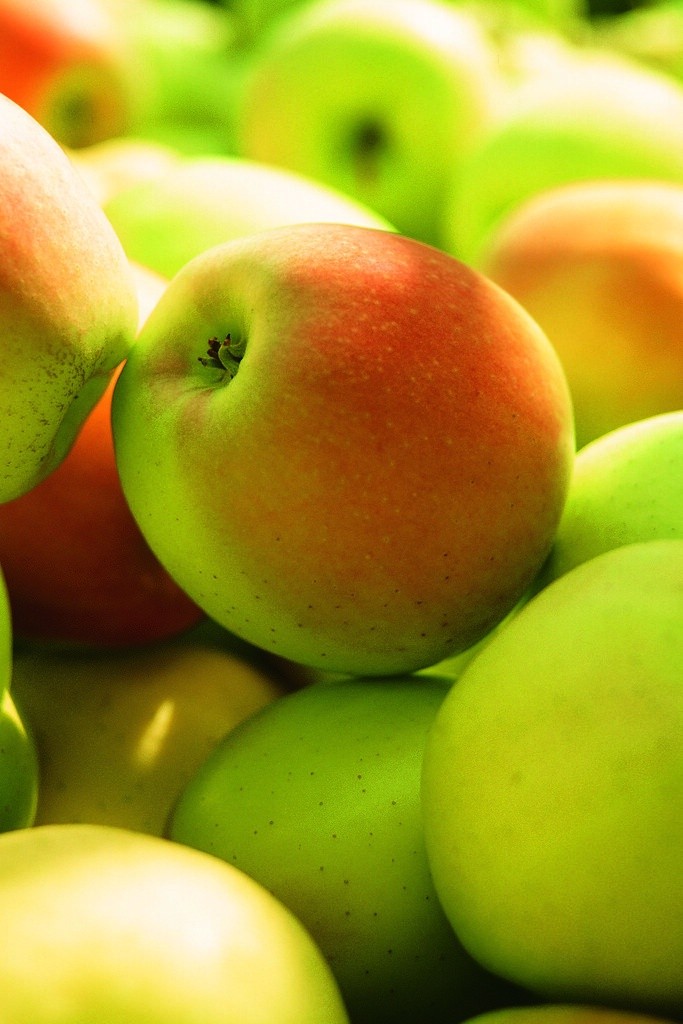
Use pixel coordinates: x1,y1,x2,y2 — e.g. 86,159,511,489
0,0,683,1024
